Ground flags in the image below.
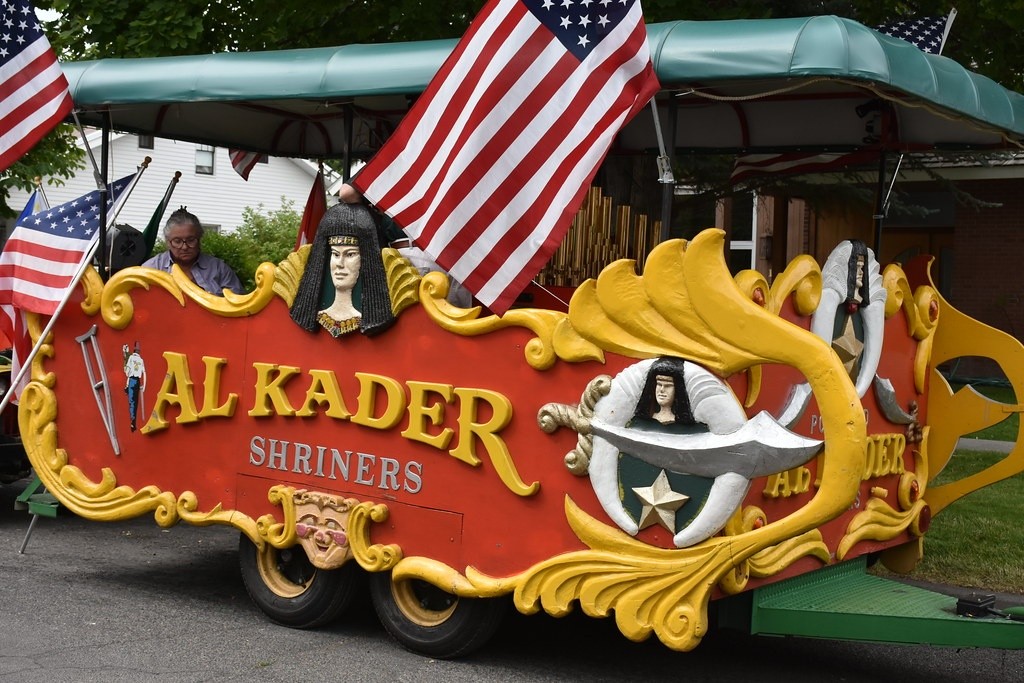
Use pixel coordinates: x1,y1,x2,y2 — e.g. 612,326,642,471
228,148,261,181
0,172,139,407
357,0,663,320
0,0,73,174
293,172,326,252
141,183,171,259
870,11,951,55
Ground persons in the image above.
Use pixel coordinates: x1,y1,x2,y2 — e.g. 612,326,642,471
287,203,394,337
634,354,693,424
142,207,245,298
339,164,472,309
846,243,871,306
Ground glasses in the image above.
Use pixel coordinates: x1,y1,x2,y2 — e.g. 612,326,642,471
168,235,200,249
